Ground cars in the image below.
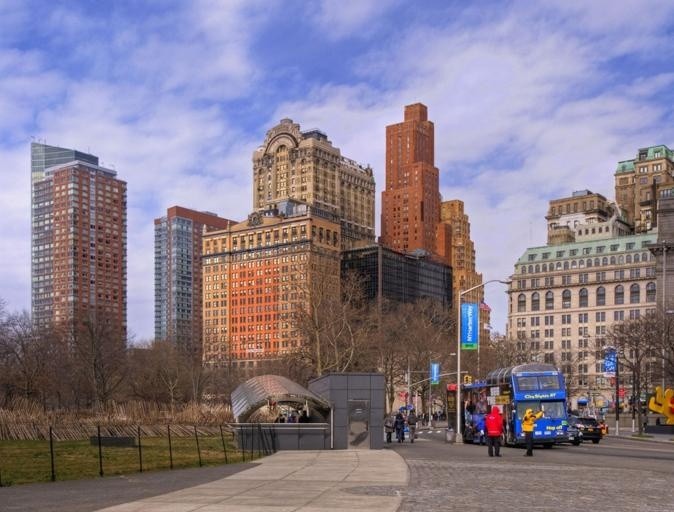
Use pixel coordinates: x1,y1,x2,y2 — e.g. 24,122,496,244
569,415,602,446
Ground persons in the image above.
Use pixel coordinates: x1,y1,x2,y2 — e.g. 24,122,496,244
394,413,405,443
485,406,507,457
384,412,393,442
408,410,418,444
522,408,545,457
276,410,313,424
419,409,446,425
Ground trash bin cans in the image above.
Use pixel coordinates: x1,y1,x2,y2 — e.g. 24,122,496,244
445,426,456,442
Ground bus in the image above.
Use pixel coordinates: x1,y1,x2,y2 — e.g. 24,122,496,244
446,363,574,447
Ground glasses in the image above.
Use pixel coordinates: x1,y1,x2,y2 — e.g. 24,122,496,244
528,410,534,414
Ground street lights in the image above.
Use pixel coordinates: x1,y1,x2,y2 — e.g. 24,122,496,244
455,279,512,444
